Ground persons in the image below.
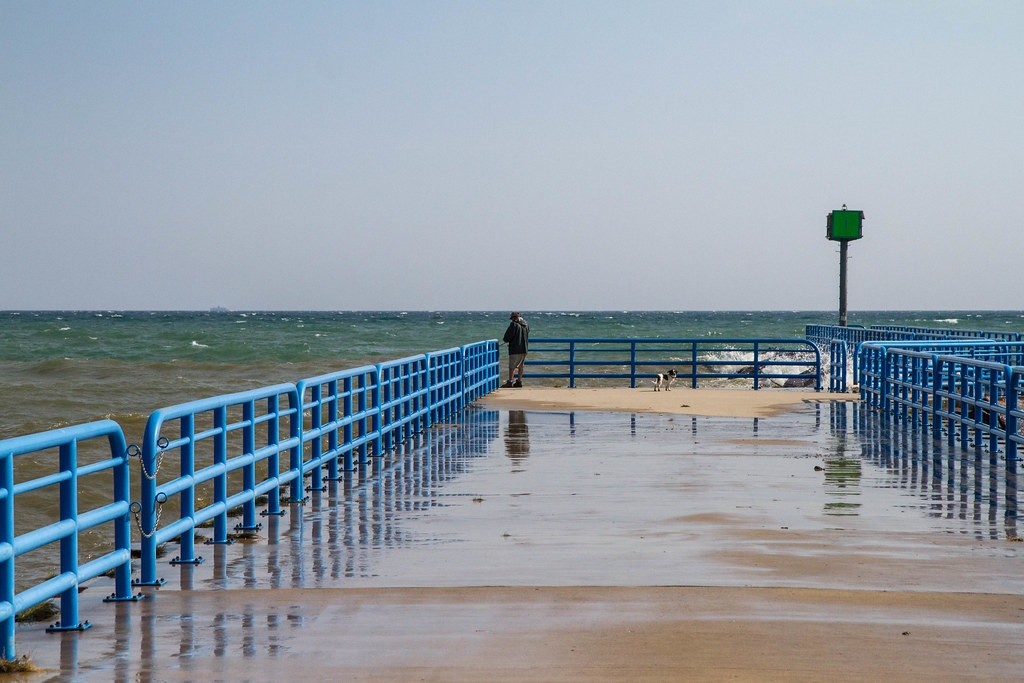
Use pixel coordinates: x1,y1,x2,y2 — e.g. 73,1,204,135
503,409,531,470
503,311,530,389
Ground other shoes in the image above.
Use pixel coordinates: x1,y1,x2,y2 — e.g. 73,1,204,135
513,380,522,387
502,380,512,387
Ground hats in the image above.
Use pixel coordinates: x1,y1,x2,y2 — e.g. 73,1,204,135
510,312,521,319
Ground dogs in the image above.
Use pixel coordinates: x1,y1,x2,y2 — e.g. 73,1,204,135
650,368,679,391
